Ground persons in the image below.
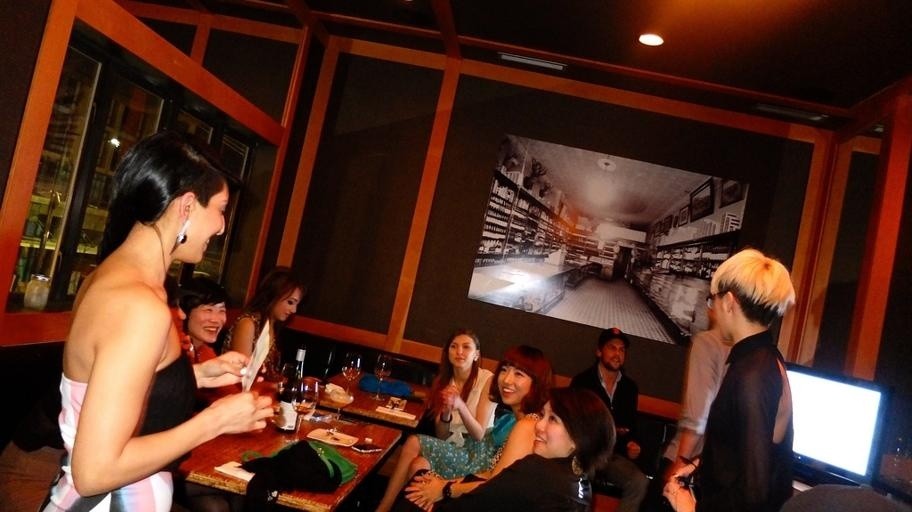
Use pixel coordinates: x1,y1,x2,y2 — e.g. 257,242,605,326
370,329,500,512
40,128,275,512
179,272,227,363
160,277,191,357
569,328,649,512
651,300,734,488
435,387,615,512
403,345,552,512
219,264,307,413
664,247,796,512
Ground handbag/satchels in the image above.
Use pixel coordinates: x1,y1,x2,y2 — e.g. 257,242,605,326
359,372,412,398
239,440,357,493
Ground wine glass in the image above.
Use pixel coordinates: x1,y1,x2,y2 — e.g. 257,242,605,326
286,379,317,443
371,354,392,401
278,362,294,395
326,388,354,434
339,352,362,404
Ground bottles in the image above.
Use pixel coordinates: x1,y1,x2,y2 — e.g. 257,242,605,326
276,345,307,433
318,350,338,380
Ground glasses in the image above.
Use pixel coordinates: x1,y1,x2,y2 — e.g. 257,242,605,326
706,290,730,310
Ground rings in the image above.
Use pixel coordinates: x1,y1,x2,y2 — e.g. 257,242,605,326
671,489,680,496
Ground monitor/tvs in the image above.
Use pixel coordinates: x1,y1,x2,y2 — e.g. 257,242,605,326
784,363,894,487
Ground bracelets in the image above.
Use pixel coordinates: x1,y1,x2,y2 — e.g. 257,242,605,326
440,410,453,424
689,462,697,469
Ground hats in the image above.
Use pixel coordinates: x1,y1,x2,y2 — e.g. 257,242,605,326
599,328,630,348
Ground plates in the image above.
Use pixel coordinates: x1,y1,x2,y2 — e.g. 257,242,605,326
306,429,359,447
385,396,408,412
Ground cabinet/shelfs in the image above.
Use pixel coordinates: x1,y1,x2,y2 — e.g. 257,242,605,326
0,0,328,357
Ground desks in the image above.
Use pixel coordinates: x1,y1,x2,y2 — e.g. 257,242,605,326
297,370,432,445
179,400,403,512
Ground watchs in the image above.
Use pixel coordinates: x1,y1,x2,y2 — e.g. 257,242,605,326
442,481,456,499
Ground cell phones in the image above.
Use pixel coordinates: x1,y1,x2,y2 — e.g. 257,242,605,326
352,444,383,453
